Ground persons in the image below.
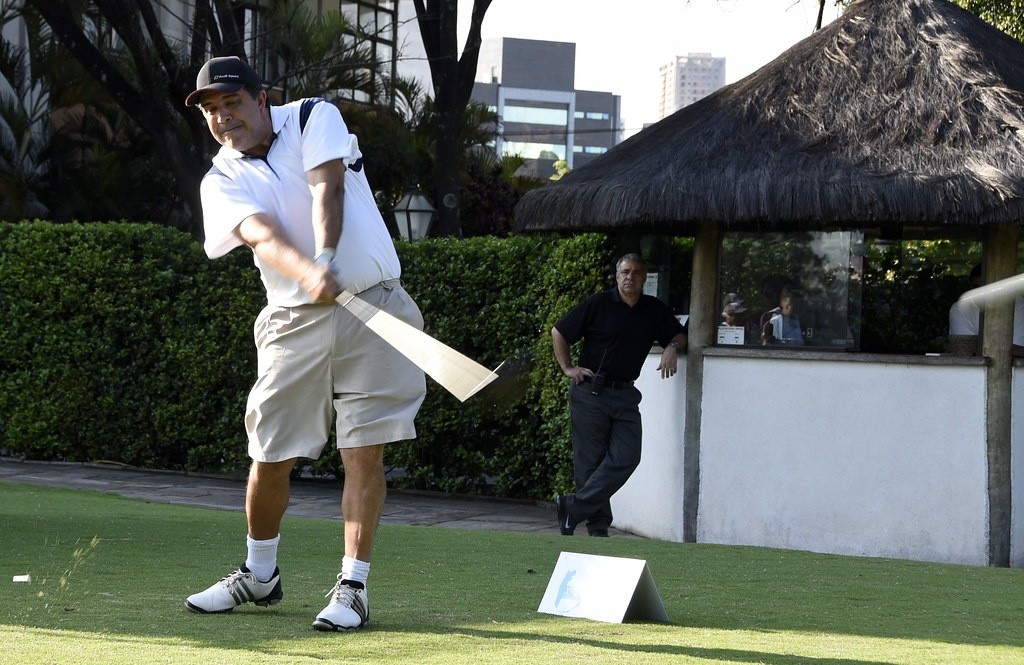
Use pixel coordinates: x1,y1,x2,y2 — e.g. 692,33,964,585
947,260,1024,362
186,58,426,630
552,252,687,536
713,289,820,346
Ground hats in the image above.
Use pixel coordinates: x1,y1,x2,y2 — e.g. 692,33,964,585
723,293,744,306
721,302,747,315
184,57,259,106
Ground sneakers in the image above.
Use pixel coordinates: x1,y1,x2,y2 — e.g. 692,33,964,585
183,562,283,614
586,521,609,537
313,571,369,631
557,493,577,536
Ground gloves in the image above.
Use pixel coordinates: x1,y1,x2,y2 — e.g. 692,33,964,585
313,249,342,289
